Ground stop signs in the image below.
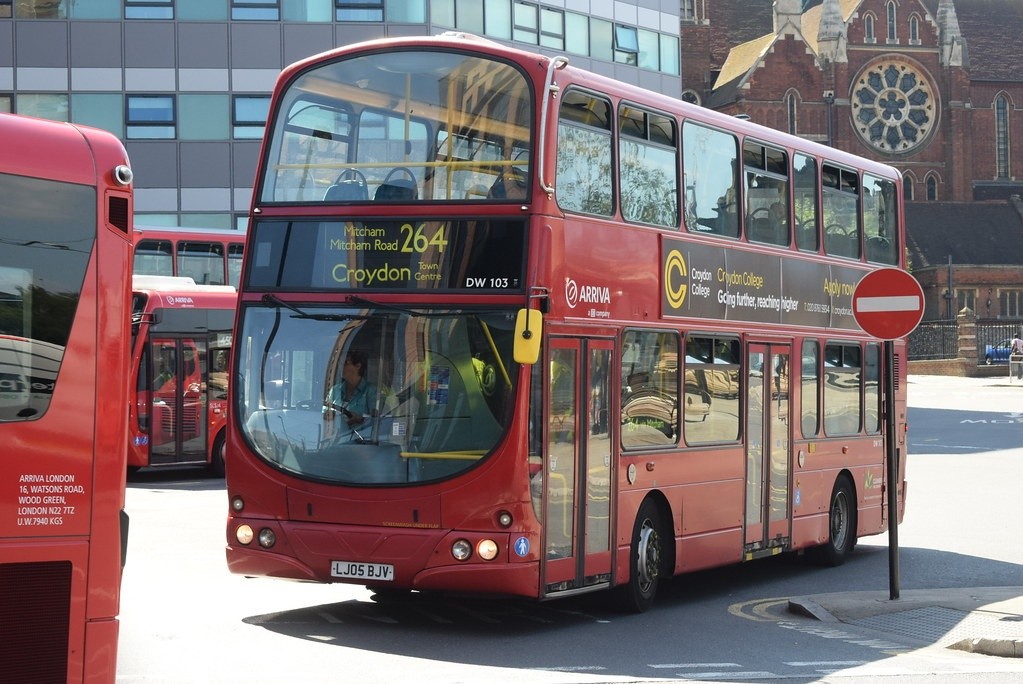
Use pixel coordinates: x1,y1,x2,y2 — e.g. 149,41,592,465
850,267,926,342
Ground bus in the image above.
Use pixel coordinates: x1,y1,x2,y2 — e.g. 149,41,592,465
1,112,134,683
225,30,908,624
129,224,247,287
127,276,237,480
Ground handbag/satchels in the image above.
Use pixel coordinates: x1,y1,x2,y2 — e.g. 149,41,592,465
1011,348,1022,361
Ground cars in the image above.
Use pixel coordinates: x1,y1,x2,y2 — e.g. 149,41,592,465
985,338,1013,365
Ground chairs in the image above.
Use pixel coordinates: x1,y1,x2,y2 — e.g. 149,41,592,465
465,168,890,265
323,168,368,201
374,167,419,201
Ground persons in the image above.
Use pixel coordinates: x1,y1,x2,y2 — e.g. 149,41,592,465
321,352,390,445
1010,333,1023,355
